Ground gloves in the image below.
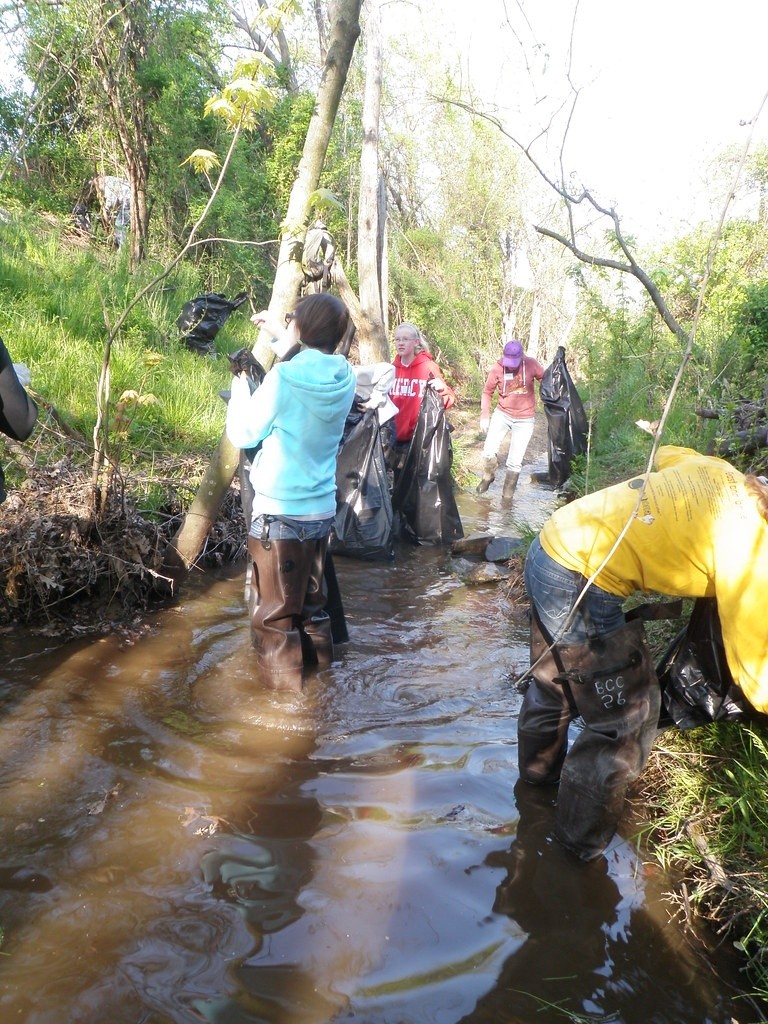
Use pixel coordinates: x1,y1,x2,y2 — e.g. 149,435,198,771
12,364,30,386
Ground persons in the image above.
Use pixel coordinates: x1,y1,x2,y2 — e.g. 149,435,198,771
0,337,37,505
225,294,356,690
349,363,400,469
386,322,456,476
476,339,546,497
82,175,131,246
516,445,767,861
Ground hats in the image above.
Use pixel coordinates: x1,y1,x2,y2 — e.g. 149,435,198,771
502,340,523,368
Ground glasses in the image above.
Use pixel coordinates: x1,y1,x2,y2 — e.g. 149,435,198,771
392,338,416,345
285,313,296,323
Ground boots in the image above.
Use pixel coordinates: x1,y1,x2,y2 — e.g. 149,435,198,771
476,464,496,493
249,539,319,690
299,536,333,665
549,619,661,861
517,600,578,787
502,471,518,499
